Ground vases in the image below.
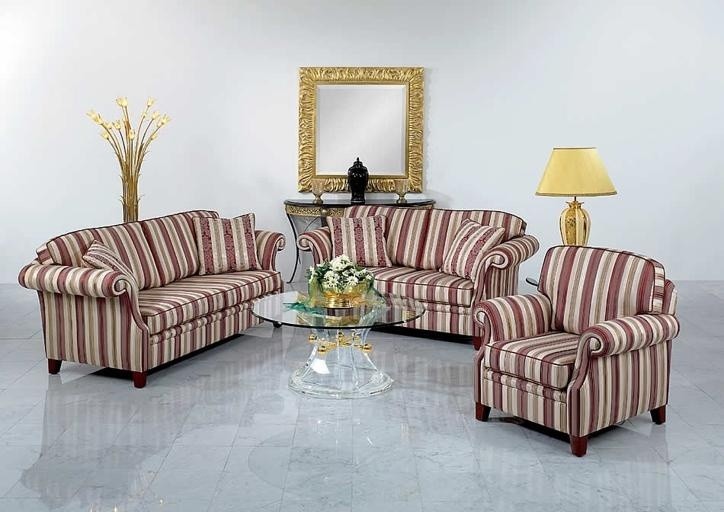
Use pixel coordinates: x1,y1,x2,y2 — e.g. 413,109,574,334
317,280,368,307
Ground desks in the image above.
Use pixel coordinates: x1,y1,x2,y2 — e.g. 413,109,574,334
282,198,437,283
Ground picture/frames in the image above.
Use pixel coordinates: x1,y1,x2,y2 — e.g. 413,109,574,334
296,65,427,194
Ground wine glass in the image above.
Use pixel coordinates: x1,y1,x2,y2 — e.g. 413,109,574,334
393,178,409,203
311,178,325,203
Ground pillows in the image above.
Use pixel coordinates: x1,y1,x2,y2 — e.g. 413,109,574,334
438,215,507,281
323,213,395,269
192,210,265,277
82,237,138,288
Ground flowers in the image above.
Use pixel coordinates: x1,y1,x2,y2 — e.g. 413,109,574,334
302,250,373,286
81,94,172,222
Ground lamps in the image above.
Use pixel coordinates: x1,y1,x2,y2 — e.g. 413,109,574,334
537,143,619,245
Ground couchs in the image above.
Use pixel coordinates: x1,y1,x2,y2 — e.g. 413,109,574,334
21,207,286,389
470,241,679,457
297,205,538,350
